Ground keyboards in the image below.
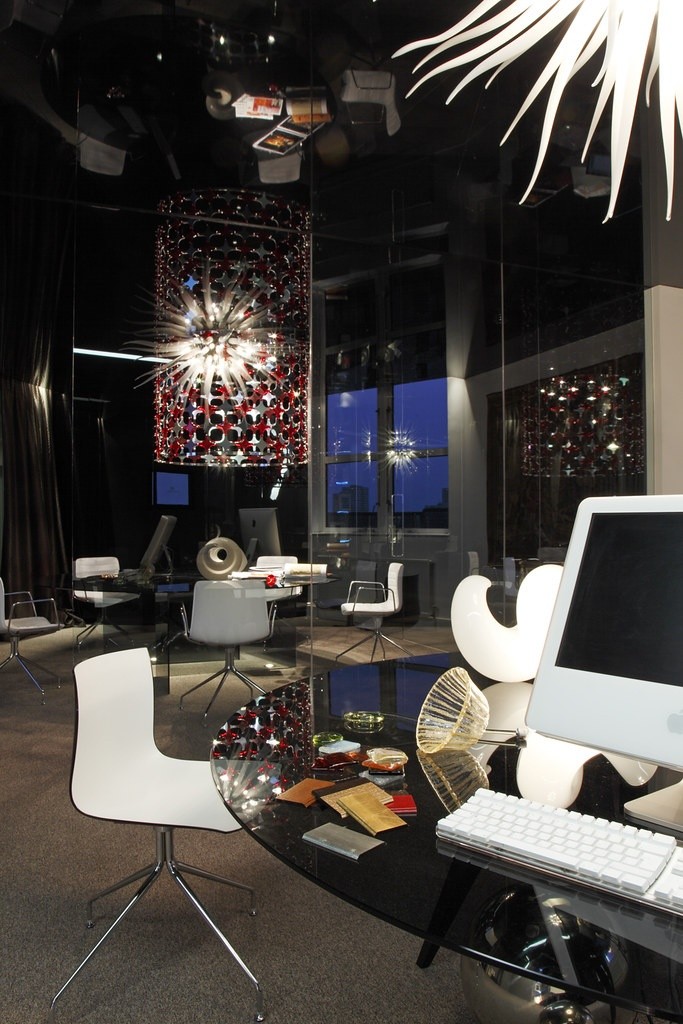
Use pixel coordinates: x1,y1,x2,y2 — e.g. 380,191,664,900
435,787,683,916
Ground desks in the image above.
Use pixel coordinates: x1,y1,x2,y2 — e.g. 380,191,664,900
51,572,339,693
209,650,682,1024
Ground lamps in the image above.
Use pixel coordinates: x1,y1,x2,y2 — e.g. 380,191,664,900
152,191,310,467
518,287,646,478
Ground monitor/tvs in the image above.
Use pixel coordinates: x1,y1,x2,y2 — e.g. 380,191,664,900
240,510,282,571
140,515,177,570
152,473,189,506
526,493,683,832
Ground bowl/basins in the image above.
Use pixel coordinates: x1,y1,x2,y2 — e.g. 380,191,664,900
416,666,490,754
415,751,488,816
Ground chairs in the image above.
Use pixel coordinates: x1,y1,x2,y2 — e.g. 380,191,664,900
74,556,134,647
334,563,414,670
0,578,65,706
48,647,281,1024
465,546,566,599
256,556,302,599
179,579,270,726
